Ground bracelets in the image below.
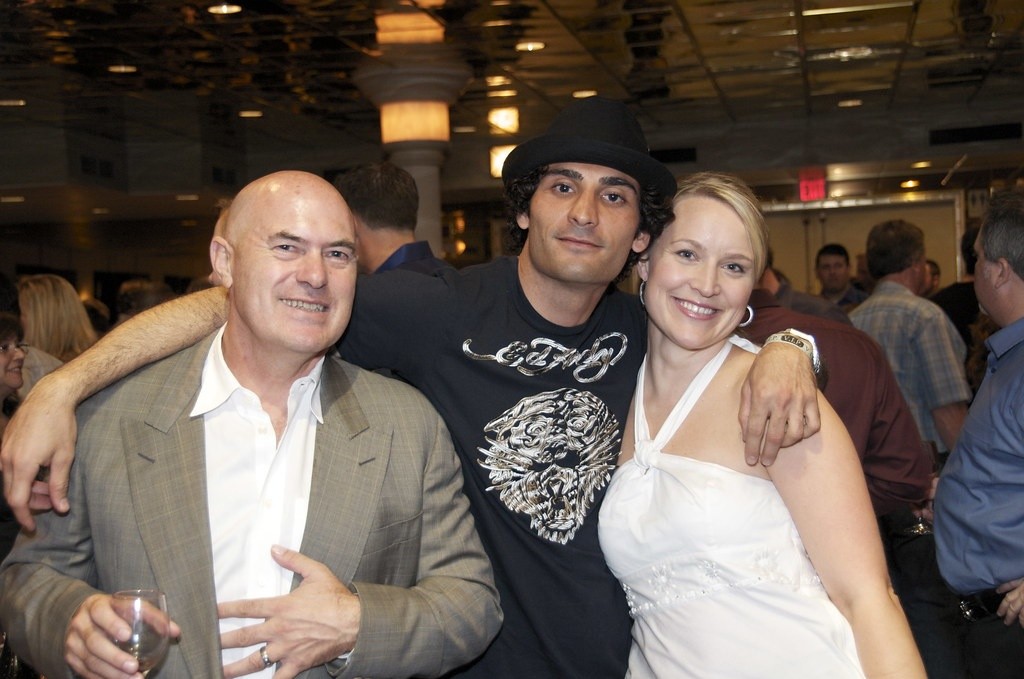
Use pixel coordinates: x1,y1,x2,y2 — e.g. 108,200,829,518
762,335,814,360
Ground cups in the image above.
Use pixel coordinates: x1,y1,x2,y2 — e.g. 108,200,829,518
105,589,171,679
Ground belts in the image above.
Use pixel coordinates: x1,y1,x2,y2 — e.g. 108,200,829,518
960,593,1005,621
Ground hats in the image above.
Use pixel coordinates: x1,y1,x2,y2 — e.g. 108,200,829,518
501,97,677,201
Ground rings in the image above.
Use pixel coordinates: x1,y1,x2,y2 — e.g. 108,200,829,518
259,645,272,667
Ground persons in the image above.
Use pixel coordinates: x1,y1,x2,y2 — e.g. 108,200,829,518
0,269,221,564
0,96,824,679
326,161,457,383
595,170,927,679
0,169,506,679
731,189,1024,679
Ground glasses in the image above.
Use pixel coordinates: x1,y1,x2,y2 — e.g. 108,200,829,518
0,343,30,354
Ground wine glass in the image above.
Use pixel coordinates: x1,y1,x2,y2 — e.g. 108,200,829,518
903,500,933,535
